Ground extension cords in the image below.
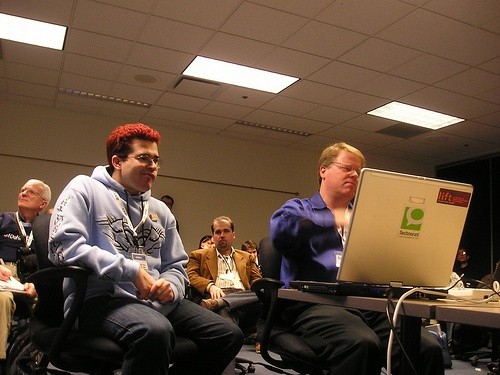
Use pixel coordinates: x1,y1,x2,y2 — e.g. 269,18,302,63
473,289,499,301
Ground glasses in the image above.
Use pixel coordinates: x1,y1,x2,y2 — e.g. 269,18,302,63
329,161,361,177
122,154,161,166
18,187,44,200
458,252,467,256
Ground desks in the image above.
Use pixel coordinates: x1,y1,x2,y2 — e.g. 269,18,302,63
277,288,500,329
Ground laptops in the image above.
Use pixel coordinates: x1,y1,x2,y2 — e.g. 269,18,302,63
289,168,473,299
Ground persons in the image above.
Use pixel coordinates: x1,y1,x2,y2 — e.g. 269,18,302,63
48,123,243,375
0,179,52,360
270,142,444,375
453,247,500,361
160,195,263,375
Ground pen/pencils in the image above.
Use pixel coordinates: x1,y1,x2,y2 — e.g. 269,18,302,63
0,258,11,281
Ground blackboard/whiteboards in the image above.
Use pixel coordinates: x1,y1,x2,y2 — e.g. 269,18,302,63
0,154,301,257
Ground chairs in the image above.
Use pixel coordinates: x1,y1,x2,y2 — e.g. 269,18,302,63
186,286,258,375
251,238,346,375
25,214,200,375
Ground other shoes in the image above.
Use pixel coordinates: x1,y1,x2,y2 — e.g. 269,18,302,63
256,341,261,353
202,299,218,310
